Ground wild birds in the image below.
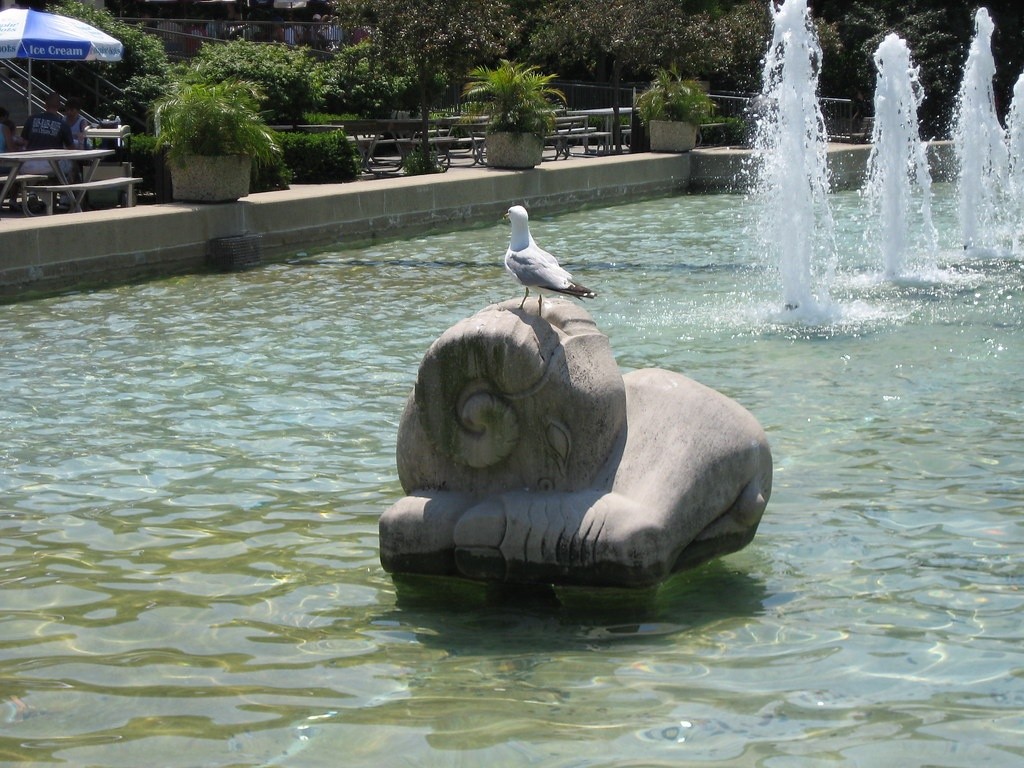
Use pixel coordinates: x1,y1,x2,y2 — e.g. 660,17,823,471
502,205,596,320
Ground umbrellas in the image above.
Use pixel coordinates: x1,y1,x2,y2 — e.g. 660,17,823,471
0,9,123,116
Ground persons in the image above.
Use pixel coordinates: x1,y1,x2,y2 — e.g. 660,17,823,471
104,0,376,65
0,91,87,209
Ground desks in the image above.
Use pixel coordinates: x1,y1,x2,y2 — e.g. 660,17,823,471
268,125,347,135
329,119,440,139
567,106,642,155
453,115,588,132
0,148,115,214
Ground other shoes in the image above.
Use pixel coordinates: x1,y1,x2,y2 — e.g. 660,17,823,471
59,194,77,207
9,200,22,211
29,194,41,205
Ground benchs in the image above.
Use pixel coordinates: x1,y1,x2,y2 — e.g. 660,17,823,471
0,174,49,205
25,176,143,216
350,126,632,179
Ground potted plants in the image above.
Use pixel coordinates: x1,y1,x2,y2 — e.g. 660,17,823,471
634,62,719,152
462,58,568,170
143,69,288,202
